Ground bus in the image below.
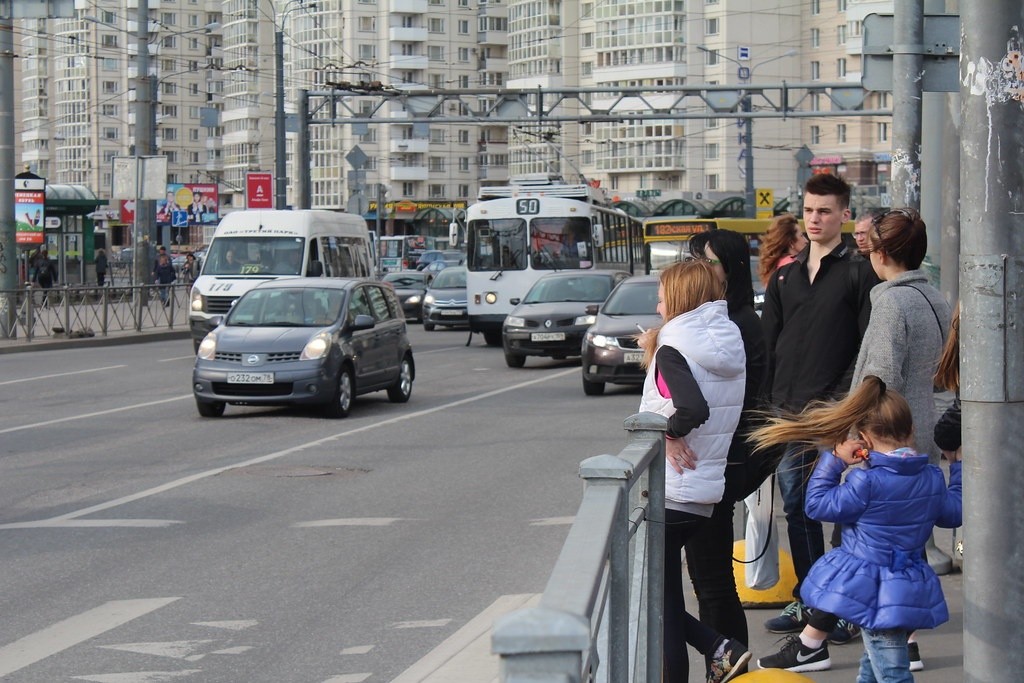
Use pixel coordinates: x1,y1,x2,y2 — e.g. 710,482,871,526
463,184,649,347
372,235,438,272
640,215,868,254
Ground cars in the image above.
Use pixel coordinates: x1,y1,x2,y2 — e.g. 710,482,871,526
420,268,476,333
115,248,203,272
579,270,668,396
500,270,636,367
749,258,768,314
381,271,433,323
191,277,416,419
422,260,462,278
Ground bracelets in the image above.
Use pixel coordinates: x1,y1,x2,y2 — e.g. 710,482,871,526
666,437,677,440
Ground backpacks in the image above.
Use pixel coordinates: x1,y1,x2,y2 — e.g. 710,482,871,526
39,263,50,276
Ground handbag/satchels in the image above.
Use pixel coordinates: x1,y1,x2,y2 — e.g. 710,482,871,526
745,475,780,589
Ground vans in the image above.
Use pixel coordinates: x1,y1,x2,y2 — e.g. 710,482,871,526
189,202,376,354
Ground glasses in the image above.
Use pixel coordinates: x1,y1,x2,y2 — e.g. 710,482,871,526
871,210,904,241
852,231,869,238
685,256,720,265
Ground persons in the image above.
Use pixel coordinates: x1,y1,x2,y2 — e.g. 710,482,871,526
638,173,962,683
94,248,109,286
273,248,302,274
159,191,181,213
182,253,200,297
560,229,578,255
152,245,175,305
405,237,415,251
221,250,240,270
30,248,58,307
188,192,217,224
319,294,354,322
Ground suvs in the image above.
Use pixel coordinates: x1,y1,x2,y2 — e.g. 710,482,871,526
417,249,461,270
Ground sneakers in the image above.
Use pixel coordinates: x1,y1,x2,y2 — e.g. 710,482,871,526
827,618,862,644
756,633,831,672
764,601,814,633
907,642,924,671
706,638,752,683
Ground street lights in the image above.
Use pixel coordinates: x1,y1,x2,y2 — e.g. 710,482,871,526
699,46,798,218
80,16,222,285
